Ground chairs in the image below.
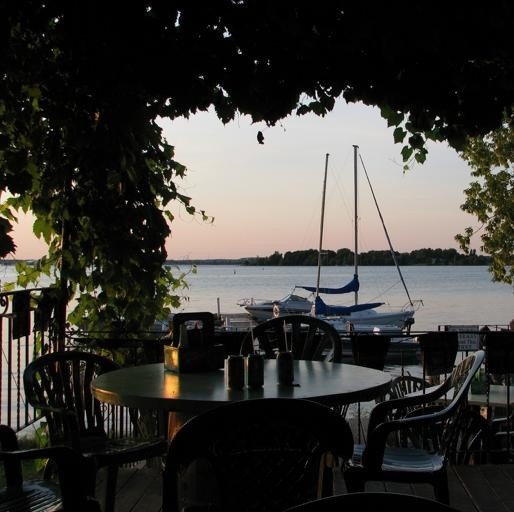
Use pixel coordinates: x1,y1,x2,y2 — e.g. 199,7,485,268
162,398,355,512
288,491,454,512
341,350,495,506
0,425,101,512
240,314,342,362
24,350,165,512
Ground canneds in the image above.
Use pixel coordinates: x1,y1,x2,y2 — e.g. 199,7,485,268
276,351,294,386
223,355,245,390
248,353,264,387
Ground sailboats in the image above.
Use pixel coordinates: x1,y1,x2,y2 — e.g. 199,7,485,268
245,145,414,335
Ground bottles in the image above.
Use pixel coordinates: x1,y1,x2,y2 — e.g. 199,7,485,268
224,351,294,389
